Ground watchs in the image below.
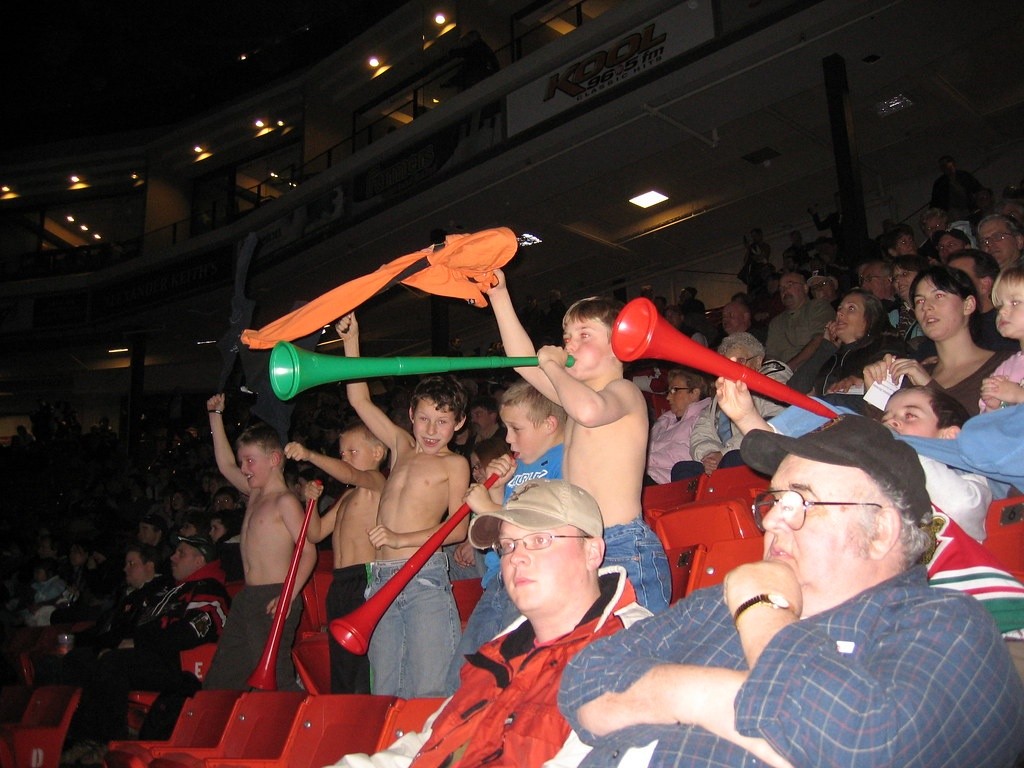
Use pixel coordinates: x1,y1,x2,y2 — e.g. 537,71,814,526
733,593,798,633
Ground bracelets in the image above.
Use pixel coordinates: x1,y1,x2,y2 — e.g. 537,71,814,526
208,410,223,414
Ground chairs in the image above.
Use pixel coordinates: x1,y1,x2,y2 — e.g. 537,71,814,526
0,464,772,768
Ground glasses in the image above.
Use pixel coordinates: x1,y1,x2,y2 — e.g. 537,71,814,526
751,490,890,532
664,385,694,398
471,463,481,474
737,356,757,365
809,279,829,292
979,233,1018,248
490,531,592,556
858,274,890,287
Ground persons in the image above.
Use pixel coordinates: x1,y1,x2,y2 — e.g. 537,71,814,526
978,268,1024,412
558,414,1024,768
624,158,1024,483
328,478,654,768
882,385,993,546
475,268,672,616
284,427,388,695
203,394,318,690
1,326,561,768
864,265,1016,416
336,311,470,700
442,383,567,697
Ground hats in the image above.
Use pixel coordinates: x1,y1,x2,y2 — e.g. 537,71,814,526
467,482,605,550
141,514,168,535
740,413,936,563
177,535,216,563
807,267,840,285
932,229,970,246
